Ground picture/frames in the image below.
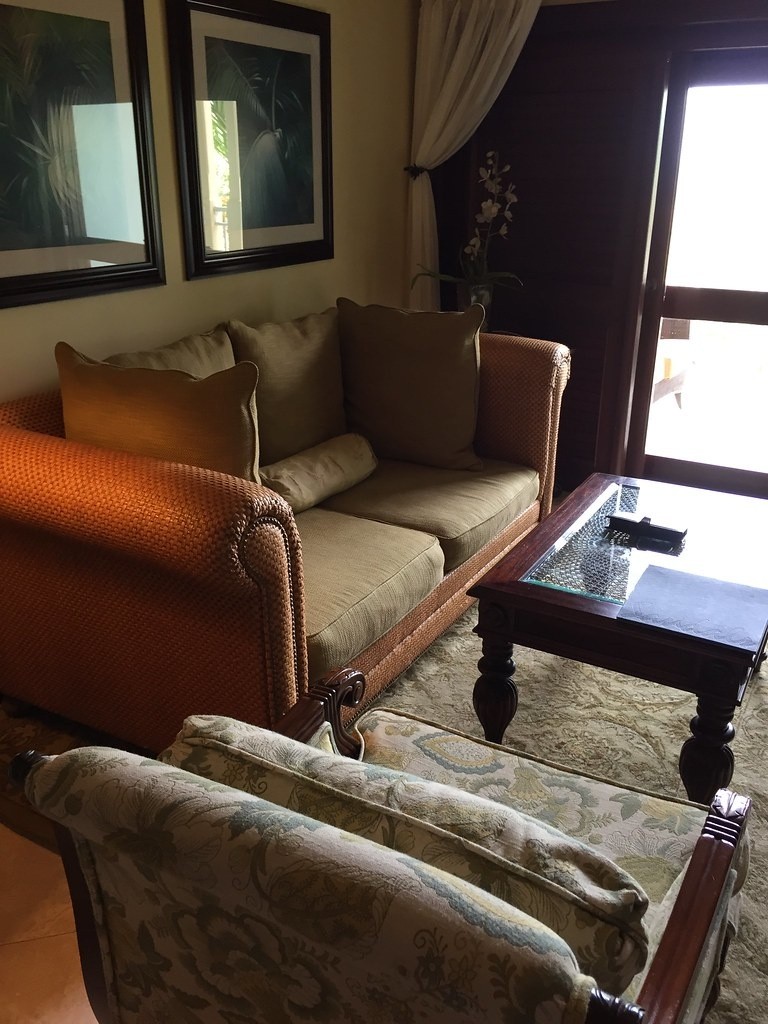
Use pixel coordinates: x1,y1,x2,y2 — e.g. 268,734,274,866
0,0,167,310
164,0,336,282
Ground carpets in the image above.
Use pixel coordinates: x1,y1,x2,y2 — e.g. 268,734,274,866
0,598,768,1022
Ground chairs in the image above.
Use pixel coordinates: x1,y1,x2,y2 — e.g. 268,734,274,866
0,666,752,1024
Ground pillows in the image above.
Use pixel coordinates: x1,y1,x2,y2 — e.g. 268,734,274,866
157,715,650,997
259,433,379,516
227,307,348,466
101,321,236,378
54,341,263,485
336,296,483,471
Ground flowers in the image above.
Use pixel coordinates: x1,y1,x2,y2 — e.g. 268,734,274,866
411,151,523,291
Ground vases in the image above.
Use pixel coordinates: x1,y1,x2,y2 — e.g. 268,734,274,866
456,283,493,333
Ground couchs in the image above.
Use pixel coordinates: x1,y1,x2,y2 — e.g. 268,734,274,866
0,333,571,754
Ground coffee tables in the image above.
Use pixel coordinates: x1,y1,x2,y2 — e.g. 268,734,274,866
465,472,768,807
